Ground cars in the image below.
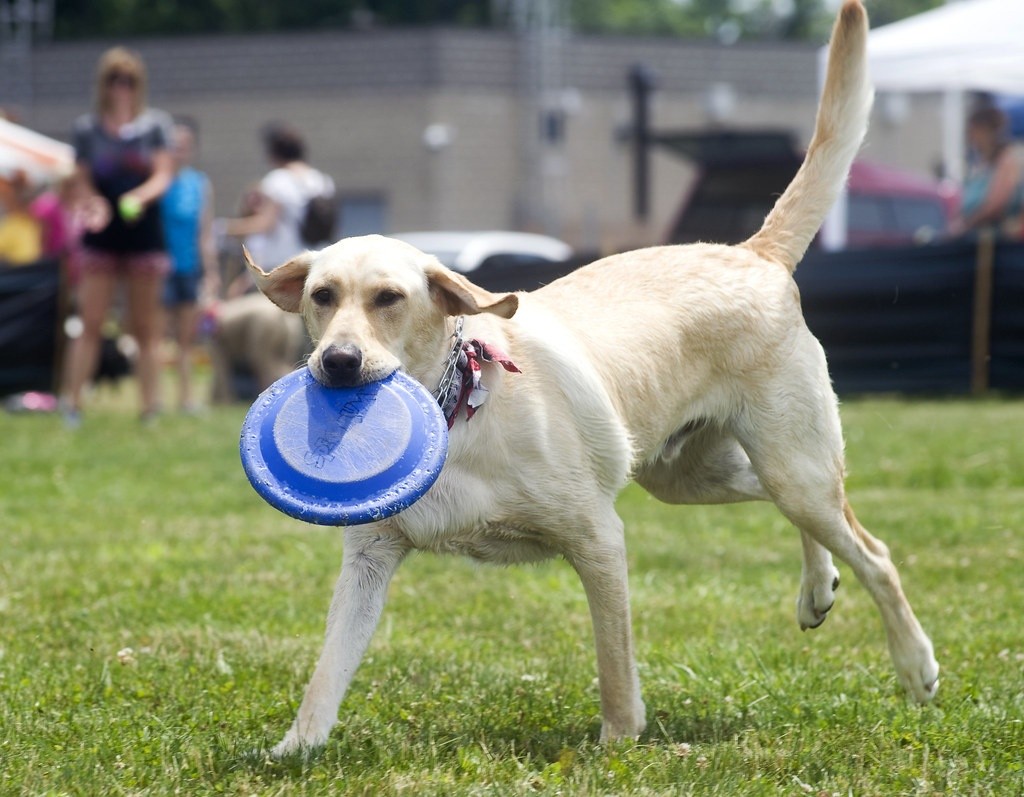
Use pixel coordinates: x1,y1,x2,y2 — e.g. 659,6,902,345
378,231,573,279
815,155,964,247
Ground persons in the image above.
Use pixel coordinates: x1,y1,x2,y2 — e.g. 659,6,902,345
154,109,218,419
58,45,176,427
211,119,339,402
946,105,1024,244
1,165,80,271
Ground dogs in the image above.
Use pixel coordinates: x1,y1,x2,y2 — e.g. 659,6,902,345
178,292,302,406
253,1,944,766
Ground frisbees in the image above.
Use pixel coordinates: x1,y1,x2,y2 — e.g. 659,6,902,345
238,363,448,528
